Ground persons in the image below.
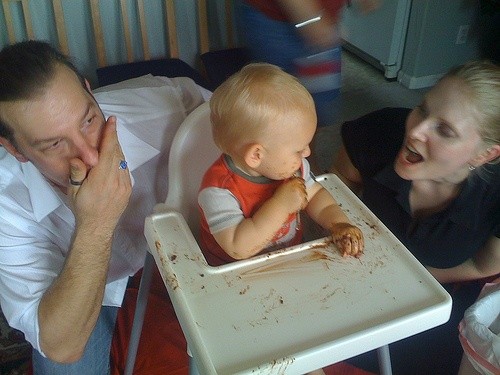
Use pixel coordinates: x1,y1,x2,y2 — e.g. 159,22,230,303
231,0,385,180
195,63,365,267
0,40,214,375
329,59,500,375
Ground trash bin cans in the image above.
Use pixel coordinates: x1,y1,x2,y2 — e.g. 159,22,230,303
457,278,500,375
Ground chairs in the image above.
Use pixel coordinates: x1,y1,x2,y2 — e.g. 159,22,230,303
124,103,453,375
1,0,249,92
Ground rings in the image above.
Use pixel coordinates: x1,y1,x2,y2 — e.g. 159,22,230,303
68,176,82,185
118,160,127,170
